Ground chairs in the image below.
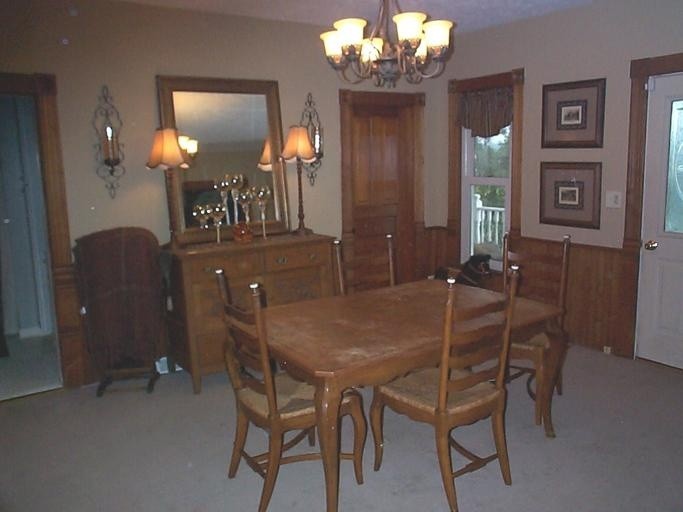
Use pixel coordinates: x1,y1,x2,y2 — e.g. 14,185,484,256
72,228,172,397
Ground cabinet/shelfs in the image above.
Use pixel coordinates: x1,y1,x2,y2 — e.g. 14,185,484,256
164,233,337,394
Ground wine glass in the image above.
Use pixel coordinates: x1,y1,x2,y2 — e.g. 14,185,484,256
193,173,272,249
193,176,254,229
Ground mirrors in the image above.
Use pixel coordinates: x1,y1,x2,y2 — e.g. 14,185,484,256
156,75,290,247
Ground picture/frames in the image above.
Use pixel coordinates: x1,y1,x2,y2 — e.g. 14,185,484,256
539,162,602,230
542,78,606,149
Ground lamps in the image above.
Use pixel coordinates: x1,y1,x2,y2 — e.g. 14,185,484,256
300,93,324,185
178,134,198,158
92,87,126,198
320,0,453,89
145,128,191,250
257,139,273,172
277,124,317,236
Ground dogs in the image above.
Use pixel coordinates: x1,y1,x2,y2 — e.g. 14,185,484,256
434,253,493,288
